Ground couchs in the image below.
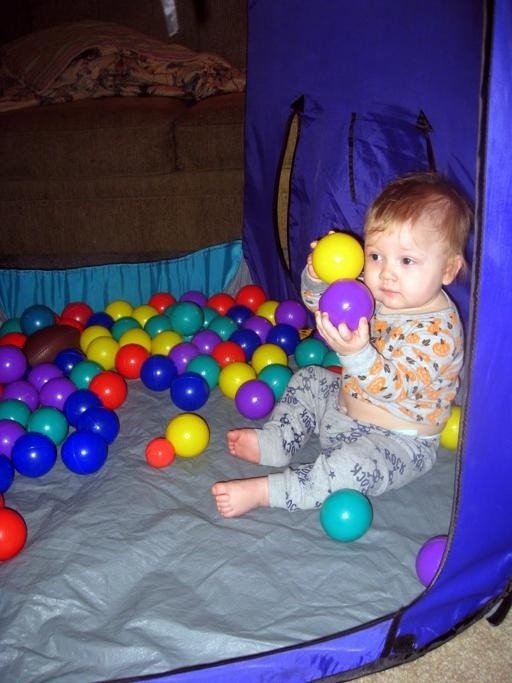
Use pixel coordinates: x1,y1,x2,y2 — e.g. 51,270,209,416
0,0,247,268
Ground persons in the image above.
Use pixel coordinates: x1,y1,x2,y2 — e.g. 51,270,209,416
211,174,472,518
